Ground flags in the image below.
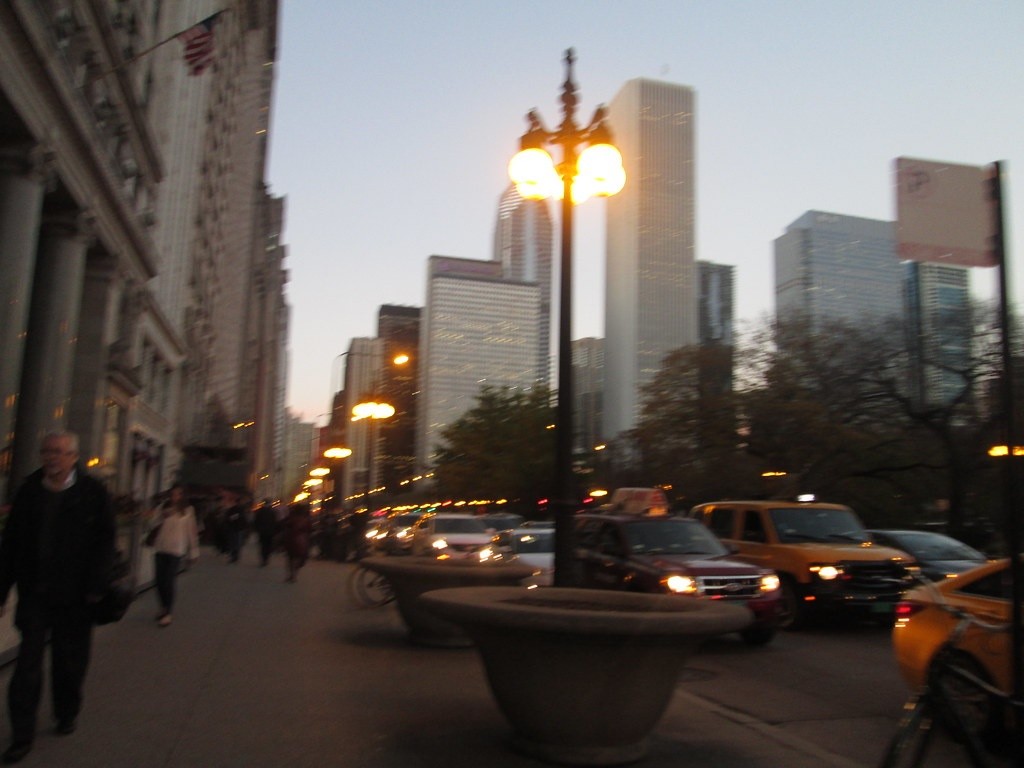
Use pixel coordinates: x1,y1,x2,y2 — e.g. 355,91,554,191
176,14,216,76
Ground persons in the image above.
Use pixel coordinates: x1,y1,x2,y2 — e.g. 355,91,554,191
151,484,200,627
0,429,117,764
209,495,368,583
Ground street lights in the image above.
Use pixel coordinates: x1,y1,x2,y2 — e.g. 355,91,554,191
508,42,625,583
293,354,410,512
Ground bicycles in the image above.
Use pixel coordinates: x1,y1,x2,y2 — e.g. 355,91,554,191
878,567,1022,768
346,544,407,608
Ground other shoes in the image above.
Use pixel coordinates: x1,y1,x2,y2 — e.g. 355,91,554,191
158,614,172,627
3,742,32,762
58,716,77,734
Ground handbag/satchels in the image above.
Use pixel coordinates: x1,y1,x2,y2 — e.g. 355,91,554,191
91,578,136,625
145,526,160,546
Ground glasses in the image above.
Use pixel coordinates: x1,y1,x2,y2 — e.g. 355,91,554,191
40,448,68,456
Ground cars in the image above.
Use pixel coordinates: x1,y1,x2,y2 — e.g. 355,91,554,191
895,556,1017,740
414,516,496,559
494,529,557,578
837,529,991,583
485,513,553,530
365,513,420,555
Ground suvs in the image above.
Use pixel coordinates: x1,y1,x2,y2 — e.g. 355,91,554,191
564,512,785,646
689,501,916,630
